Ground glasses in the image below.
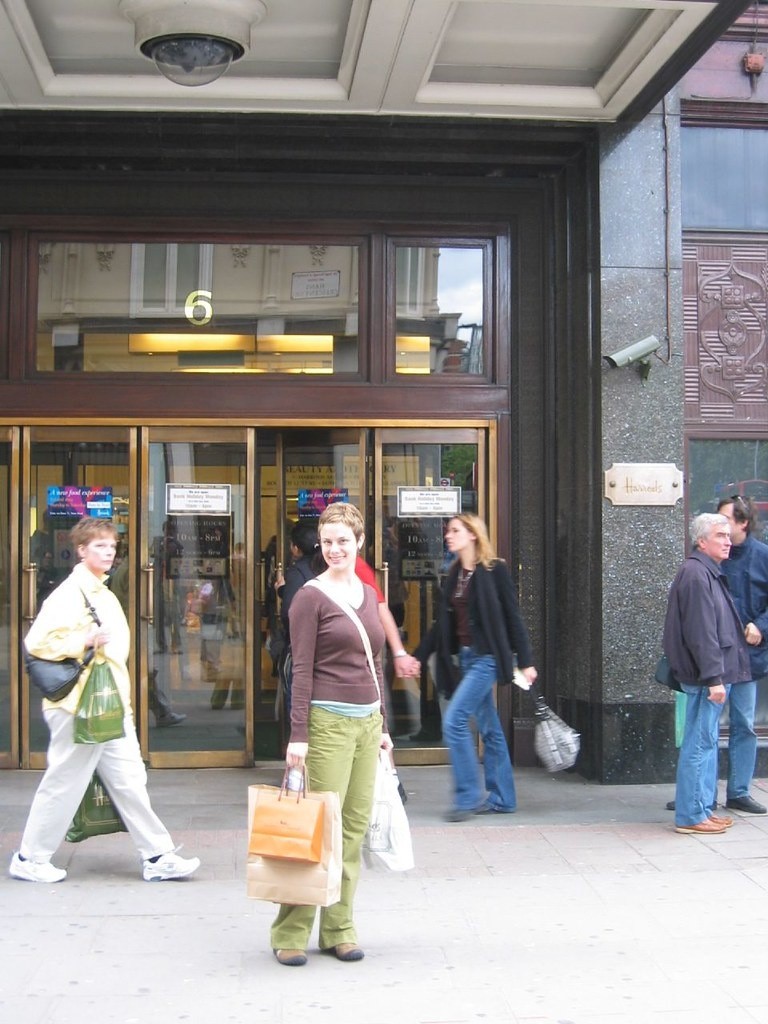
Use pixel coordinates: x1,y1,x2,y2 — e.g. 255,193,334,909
732,495,750,511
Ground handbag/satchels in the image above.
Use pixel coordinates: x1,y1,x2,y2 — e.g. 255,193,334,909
74,647,126,745
530,682,580,771
246,764,342,907
655,654,684,693
249,765,324,862
65,760,150,842
201,614,246,682
361,750,415,872
23,589,102,702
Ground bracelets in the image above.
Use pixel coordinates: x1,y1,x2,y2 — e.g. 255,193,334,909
394,649,406,659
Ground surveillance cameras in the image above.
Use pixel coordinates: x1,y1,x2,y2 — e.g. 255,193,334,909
603,334,661,370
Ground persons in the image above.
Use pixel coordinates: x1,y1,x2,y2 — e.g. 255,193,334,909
404,512,538,824
150,522,183,654
270,502,393,965
354,555,416,679
9,516,202,884
667,494,768,813
664,513,752,835
274,518,329,629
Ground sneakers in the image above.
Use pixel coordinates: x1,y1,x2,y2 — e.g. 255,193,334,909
10,852,67,882
155,712,186,728
144,843,201,881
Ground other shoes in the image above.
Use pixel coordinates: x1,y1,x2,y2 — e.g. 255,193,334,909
708,814,733,827
273,948,307,966
726,795,767,814
445,804,488,822
409,731,442,742
476,804,498,815
675,818,727,834
333,943,364,960
666,801,718,810
